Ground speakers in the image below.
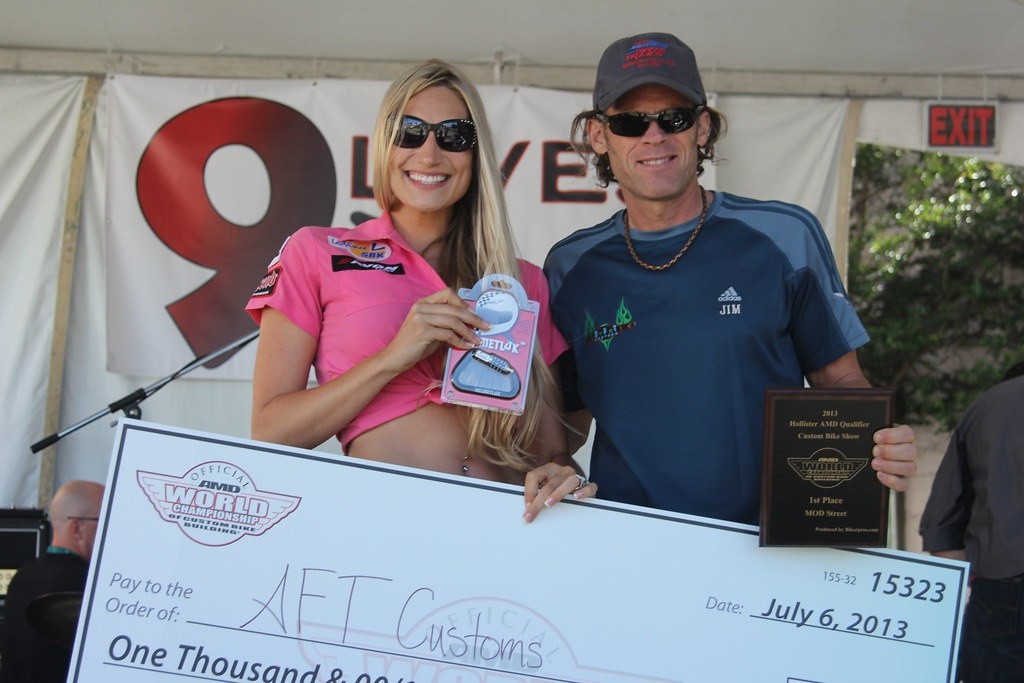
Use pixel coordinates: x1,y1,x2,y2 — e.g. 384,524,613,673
0,517,52,608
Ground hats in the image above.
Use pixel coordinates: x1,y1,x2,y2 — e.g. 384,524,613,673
592,32,707,112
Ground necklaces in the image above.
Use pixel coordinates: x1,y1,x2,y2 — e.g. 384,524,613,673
624,183,707,271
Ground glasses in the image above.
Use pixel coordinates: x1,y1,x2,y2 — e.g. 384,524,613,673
386,115,478,153
593,105,704,138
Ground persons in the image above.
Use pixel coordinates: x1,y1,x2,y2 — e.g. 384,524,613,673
0,481,105,683
244,58,598,524
538,30,918,528
920,376,1024,683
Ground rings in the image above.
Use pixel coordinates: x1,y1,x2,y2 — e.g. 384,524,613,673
575,474,587,490
